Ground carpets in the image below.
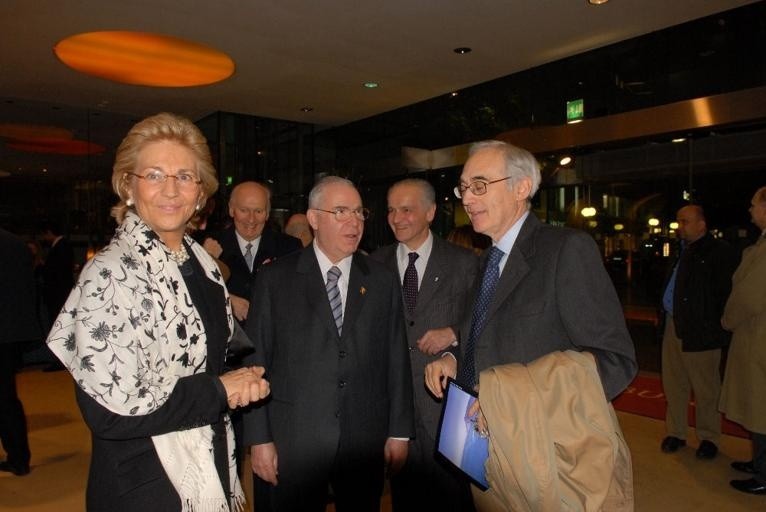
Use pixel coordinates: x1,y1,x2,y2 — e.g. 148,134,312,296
609,374,753,437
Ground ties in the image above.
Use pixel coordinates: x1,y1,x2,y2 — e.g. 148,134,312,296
460,246,505,387
244,244,253,271
325,266,342,336
403,253,419,313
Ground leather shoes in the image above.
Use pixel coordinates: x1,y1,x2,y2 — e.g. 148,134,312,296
730,478,766,494
661,437,685,451
731,461,758,472
696,439,717,458
0,459,30,476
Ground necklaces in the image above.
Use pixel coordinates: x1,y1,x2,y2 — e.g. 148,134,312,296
160,244,190,266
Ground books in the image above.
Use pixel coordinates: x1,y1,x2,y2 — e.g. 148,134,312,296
434,376,490,491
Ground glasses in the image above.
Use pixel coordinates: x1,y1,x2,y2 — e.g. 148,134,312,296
454,176,509,199
313,208,370,222
127,170,202,185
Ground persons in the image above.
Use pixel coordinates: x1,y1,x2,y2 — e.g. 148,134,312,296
369,178,481,512
661,204,732,460
242,176,415,512
46,111,272,512
186,196,244,299
0,231,47,476
222,180,290,329
720,186,766,495
35,215,75,275
23,237,43,273
282,209,314,249
423,139,639,512
